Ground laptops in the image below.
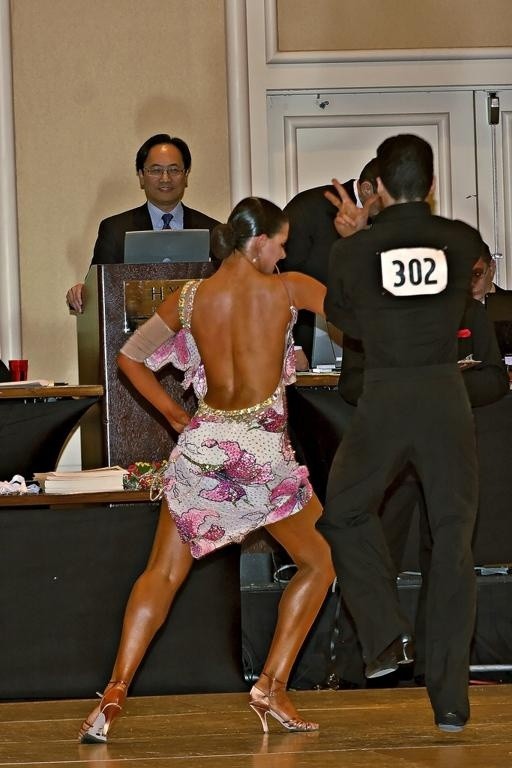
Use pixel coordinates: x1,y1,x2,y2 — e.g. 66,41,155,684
122,228,211,265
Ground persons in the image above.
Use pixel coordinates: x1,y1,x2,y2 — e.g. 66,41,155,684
338,297,508,692
471,242,512,382
77,196,337,747
65,133,224,314
322,134,491,732
274,157,382,372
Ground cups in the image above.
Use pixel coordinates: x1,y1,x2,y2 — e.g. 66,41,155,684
8,360,28,382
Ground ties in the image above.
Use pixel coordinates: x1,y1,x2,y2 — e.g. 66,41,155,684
161,213,173,229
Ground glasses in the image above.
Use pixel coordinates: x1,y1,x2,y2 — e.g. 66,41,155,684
473,262,490,280
143,165,187,177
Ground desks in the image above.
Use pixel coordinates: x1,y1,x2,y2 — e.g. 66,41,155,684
0,381,512,697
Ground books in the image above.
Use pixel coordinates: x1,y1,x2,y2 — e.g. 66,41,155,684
32,465,130,494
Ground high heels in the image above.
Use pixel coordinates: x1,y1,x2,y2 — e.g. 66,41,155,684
77,680,128,745
364,633,416,678
434,711,465,734
248,685,321,734
